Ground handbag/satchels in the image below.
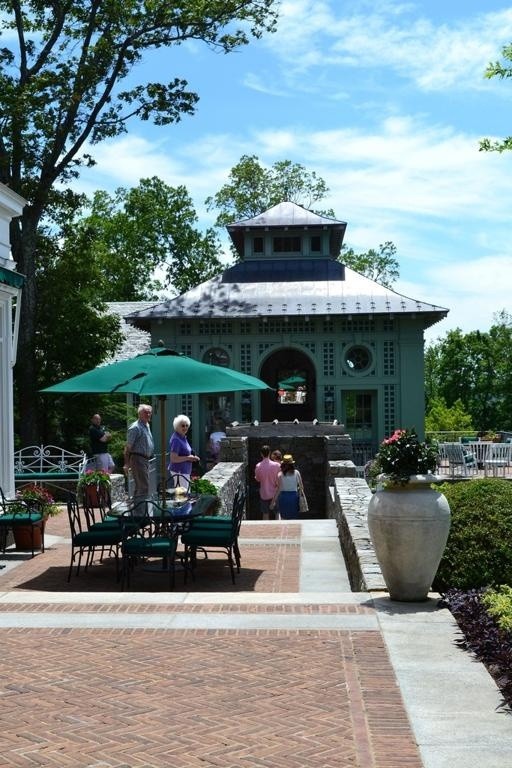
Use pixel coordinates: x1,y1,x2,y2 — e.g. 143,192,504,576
296,470,310,513
269,488,280,511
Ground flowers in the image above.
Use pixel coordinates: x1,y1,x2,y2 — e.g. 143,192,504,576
6,484,64,519
76,467,113,502
374,425,443,485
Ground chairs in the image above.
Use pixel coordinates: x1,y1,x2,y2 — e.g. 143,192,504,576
0,486,47,559
363,461,373,485
439,435,511,477
63,473,247,592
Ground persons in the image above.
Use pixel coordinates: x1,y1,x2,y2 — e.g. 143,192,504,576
123,404,156,496
88,411,116,475
269,454,304,519
169,500,193,515
210,431,227,459
167,415,201,488
254,446,281,520
132,497,149,516
295,386,305,404
271,450,282,464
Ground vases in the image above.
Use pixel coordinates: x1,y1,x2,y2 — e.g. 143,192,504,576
85,484,102,505
375,470,449,491
13,516,47,551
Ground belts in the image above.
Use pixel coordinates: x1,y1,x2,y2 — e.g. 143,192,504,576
131,453,151,461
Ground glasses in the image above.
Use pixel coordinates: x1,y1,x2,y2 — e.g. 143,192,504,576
182,424,188,428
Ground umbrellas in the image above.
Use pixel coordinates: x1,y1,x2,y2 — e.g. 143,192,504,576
278,383,296,391
36,340,273,509
280,376,305,385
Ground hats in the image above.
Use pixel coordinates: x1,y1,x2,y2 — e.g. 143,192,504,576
280,454,295,464
